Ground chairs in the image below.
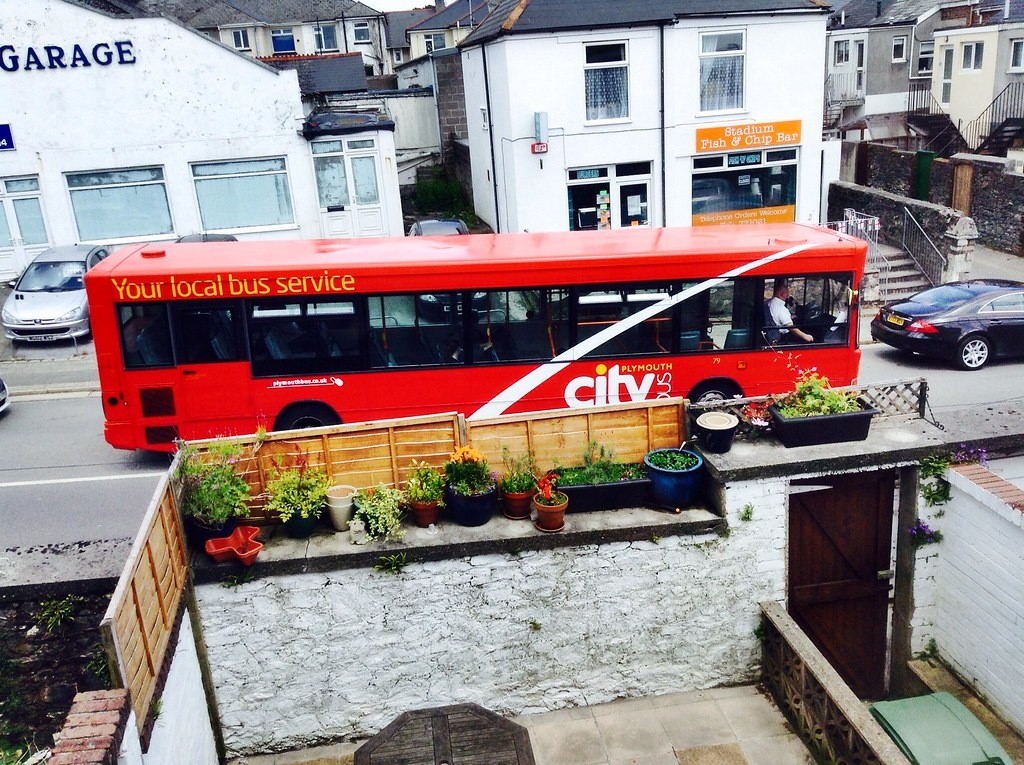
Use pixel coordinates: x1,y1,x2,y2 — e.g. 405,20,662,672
351,704,536,765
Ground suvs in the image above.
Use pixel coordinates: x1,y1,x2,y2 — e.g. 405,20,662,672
407,220,493,323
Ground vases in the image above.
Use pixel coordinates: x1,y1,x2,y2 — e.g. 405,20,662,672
327,485,357,532
445,484,498,528
354,498,390,536
767,397,881,448
534,491,568,533
191,517,236,549
407,495,440,528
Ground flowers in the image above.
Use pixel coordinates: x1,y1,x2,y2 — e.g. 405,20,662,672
171,419,267,529
760,330,864,419
351,479,406,543
536,474,566,506
445,445,500,497
405,458,445,509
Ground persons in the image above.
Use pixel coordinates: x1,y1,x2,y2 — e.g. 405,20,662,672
443,321,494,365
766,285,814,343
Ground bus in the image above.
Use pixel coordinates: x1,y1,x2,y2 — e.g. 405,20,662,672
82,221,870,452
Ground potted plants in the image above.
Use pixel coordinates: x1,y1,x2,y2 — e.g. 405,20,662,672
262,444,330,538
499,445,538,520
644,448,703,511
548,440,651,514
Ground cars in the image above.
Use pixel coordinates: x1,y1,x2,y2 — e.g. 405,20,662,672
2,242,134,343
868,279,1024,371
141,233,238,321
